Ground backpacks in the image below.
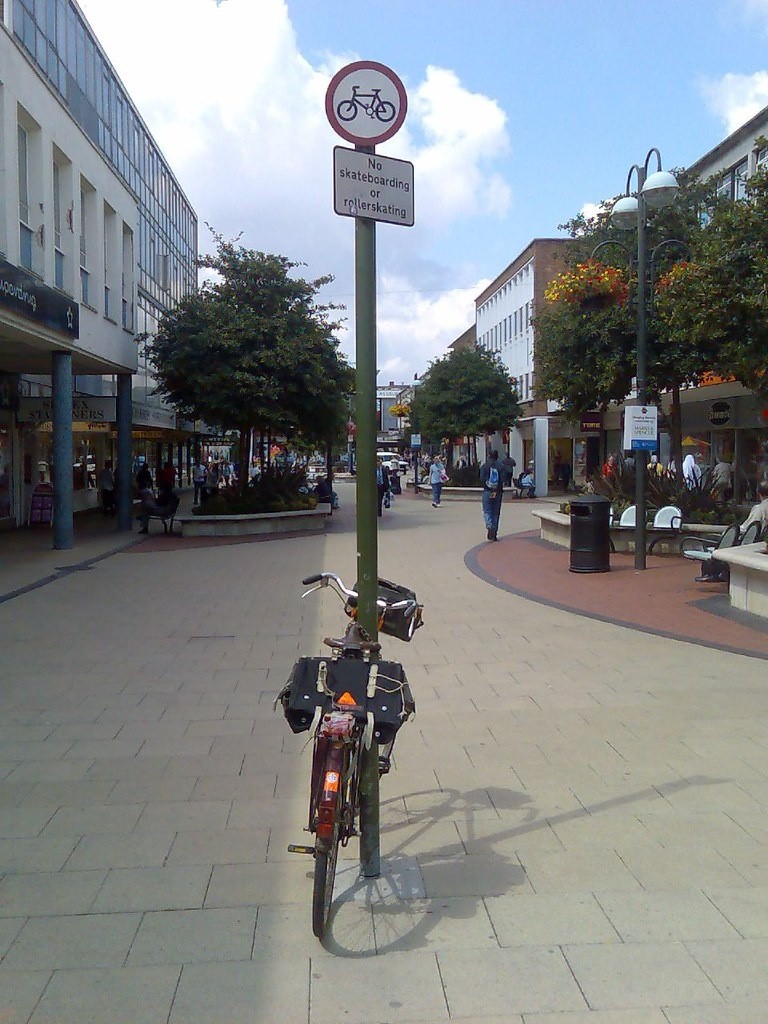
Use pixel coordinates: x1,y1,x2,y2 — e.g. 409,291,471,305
484,461,502,493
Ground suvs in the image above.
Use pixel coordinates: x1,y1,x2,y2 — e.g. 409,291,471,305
376,452,410,475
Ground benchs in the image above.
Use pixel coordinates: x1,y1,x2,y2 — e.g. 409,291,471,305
513,477,529,498
609,504,684,554
150,497,180,535
681,521,768,577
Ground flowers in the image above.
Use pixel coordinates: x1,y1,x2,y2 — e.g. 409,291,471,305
669,260,697,281
544,260,630,307
389,404,411,416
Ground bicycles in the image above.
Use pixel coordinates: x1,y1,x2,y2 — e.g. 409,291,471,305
286,572,420,939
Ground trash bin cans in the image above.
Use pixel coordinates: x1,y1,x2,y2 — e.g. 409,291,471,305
569,496,613,574
389,476,401,494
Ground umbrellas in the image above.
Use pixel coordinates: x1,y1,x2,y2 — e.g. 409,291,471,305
681,435,711,446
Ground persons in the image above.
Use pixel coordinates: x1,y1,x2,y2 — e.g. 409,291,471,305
155,462,177,489
502,452,516,486
603,455,617,478
377,460,390,516
135,463,153,490
457,456,466,468
391,456,401,477
624,453,634,466
129,481,178,533
207,455,242,488
647,453,733,497
479,450,506,541
193,458,209,504
519,470,536,498
100,461,117,517
404,447,431,471
549,458,570,492
316,476,339,510
429,456,446,507
695,480,768,582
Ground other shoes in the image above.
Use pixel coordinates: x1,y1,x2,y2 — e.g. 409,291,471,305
488,529,498,542
193,501,197,504
136,515,142,520
432,502,441,507
527,492,536,498
695,574,725,582
139,529,149,534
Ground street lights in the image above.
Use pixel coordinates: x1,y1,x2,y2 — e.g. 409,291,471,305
608,147,681,568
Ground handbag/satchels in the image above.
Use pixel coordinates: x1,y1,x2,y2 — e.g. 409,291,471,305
276,658,415,743
439,470,449,483
384,492,391,509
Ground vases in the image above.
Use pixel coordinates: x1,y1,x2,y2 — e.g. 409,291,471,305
398,412,405,417
